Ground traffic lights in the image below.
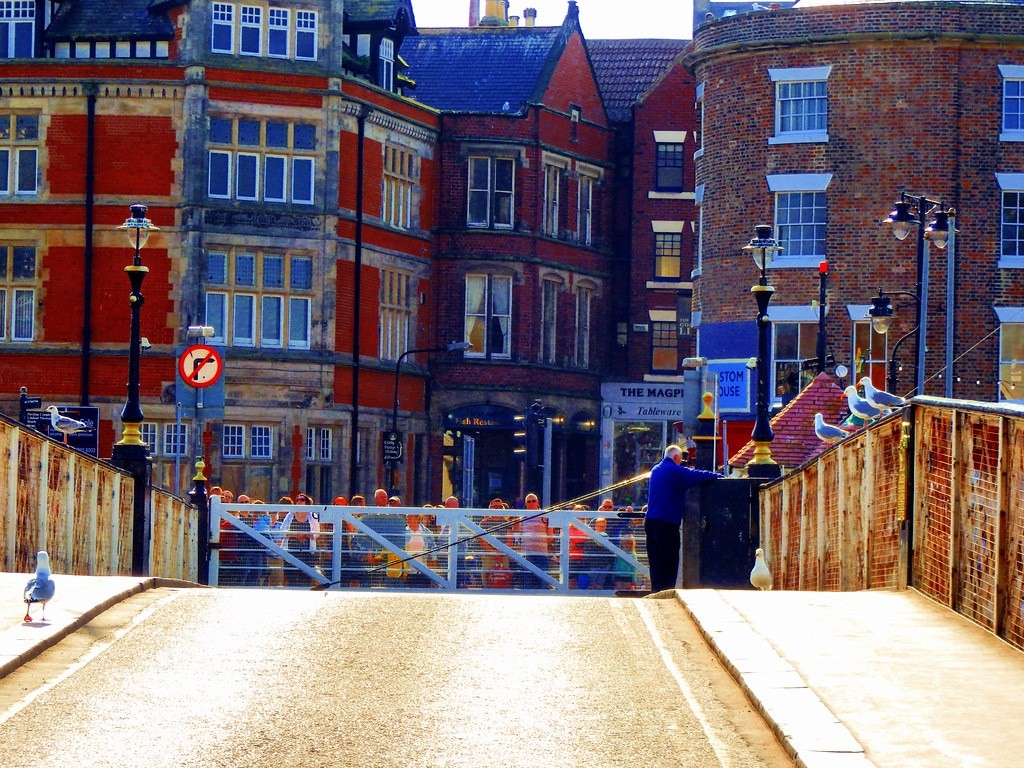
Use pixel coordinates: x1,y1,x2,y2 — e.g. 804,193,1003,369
513,406,539,467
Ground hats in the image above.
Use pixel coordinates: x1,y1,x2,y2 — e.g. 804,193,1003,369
333,497,347,505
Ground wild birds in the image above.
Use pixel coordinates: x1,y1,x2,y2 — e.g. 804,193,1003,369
854,376,906,421
23,551,55,622
750,549,773,591
840,385,892,429
811,413,850,445
42,405,94,444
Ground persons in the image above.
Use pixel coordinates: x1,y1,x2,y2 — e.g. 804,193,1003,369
643,446,726,592
209,486,649,592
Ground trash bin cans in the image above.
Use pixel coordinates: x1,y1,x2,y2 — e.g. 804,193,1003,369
681,477,774,585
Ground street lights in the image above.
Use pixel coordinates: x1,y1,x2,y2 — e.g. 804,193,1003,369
742,224,785,478
869,287,921,395
386,342,475,497
881,193,960,398
110,204,161,474
835,365,848,391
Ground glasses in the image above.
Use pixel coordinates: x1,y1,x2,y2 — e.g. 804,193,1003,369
239,500,250,504
603,505,613,509
296,498,305,502
493,506,503,509
527,500,537,503
